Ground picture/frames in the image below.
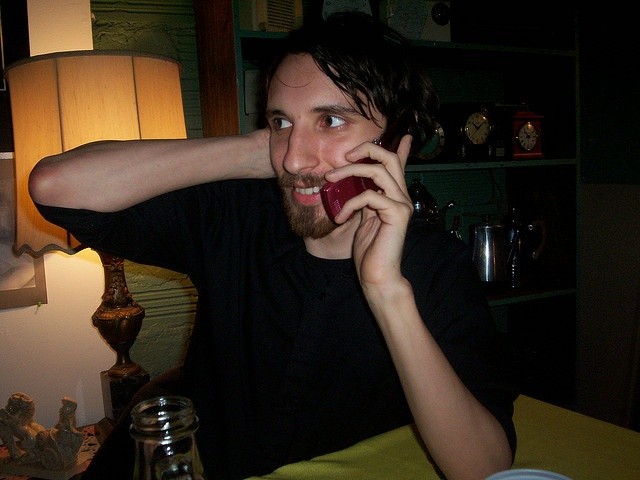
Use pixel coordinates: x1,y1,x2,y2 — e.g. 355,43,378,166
0,158,48,311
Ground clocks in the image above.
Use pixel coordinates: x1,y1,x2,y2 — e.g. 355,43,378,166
417,121,446,161
507,112,543,159
457,107,496,162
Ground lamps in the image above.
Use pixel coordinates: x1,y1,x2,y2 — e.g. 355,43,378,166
6,50,187,445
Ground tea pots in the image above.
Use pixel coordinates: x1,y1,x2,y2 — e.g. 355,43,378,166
465,211,521,295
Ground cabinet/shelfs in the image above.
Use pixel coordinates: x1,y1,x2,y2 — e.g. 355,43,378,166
196,0,583,414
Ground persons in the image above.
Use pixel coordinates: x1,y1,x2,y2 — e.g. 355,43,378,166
29,12,516,480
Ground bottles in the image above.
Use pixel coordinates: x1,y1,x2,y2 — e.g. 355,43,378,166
129,396,205,480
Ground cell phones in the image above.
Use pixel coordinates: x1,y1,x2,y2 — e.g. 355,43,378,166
319,137,395,225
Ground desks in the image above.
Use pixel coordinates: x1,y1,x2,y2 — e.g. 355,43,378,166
271,394,640,480
